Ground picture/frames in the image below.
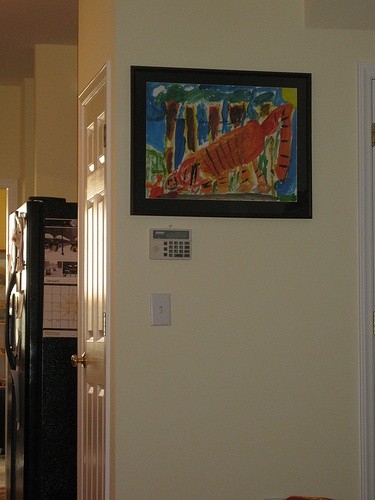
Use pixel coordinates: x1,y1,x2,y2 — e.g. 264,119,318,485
127,65,313,219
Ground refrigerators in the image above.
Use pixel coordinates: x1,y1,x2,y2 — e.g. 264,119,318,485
2,194,78,499
